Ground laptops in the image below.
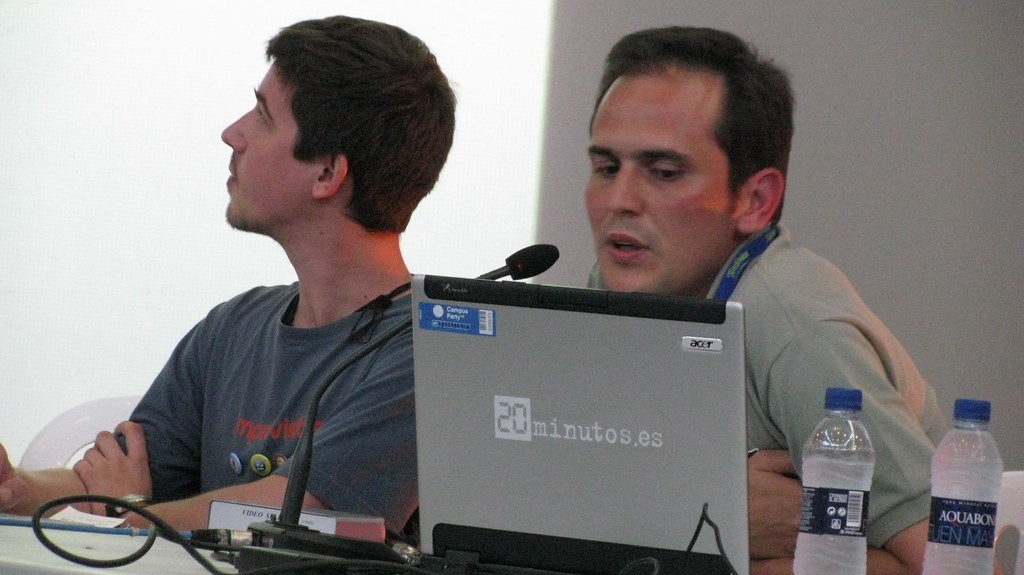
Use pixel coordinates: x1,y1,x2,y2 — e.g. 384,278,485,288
409,273,751,575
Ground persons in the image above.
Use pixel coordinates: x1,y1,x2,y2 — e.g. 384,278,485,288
0,15,457,547
584,26,950,575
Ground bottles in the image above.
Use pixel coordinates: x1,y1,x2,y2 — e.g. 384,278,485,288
790,387,875,575
922,398,1002,575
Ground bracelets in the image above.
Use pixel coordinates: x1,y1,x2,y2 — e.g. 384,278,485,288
105,494,158,517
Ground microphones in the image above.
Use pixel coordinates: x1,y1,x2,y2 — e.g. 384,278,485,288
239,244,560,575
